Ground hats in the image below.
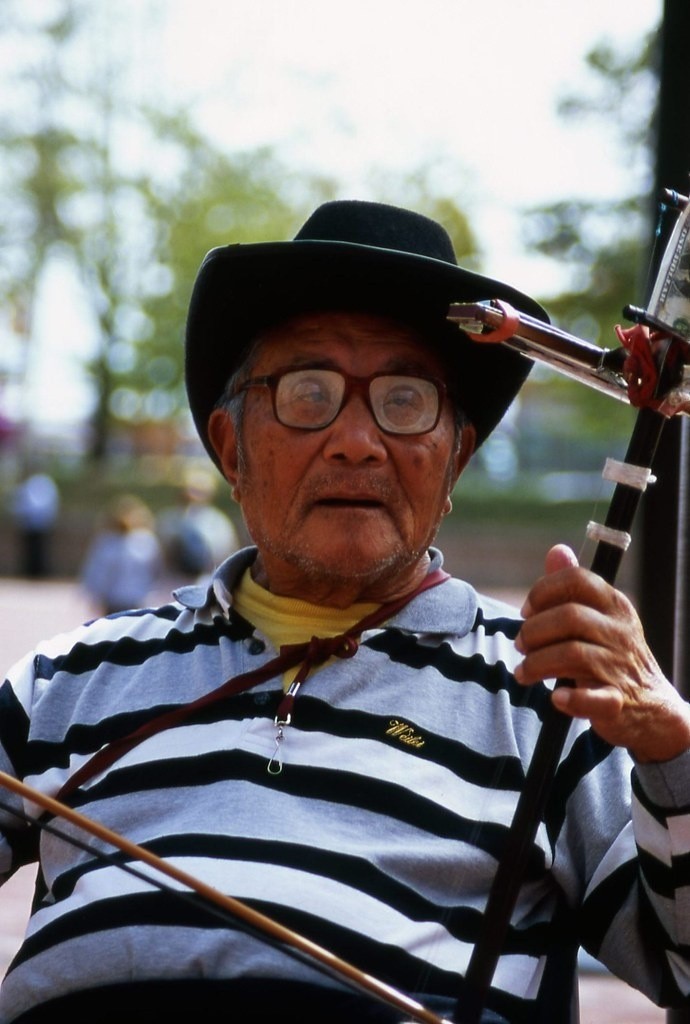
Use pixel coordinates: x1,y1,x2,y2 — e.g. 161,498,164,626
185,199,552,488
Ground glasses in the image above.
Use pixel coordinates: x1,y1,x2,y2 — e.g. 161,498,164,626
229,361,453,438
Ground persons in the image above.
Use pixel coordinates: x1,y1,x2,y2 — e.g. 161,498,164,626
17,466,60,575
81,495,159,616
169,468,238,589
0,198,690,1024
